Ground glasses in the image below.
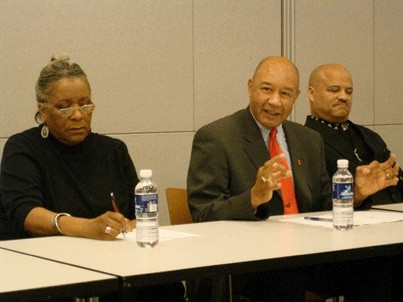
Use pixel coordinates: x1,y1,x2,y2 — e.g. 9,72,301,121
43,102,95,117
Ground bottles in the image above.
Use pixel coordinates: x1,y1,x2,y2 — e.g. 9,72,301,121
134,170,159,247
332,159,354,231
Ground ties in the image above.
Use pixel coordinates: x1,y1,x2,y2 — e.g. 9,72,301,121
269,128,298,215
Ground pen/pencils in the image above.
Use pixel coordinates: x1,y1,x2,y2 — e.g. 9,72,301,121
304,216,333,222
110,191,126,238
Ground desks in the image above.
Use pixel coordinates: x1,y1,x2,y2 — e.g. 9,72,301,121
0,208,403,302
370,202,403,213
0,248,120,302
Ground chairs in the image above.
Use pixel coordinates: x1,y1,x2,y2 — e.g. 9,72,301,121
165,187,193,226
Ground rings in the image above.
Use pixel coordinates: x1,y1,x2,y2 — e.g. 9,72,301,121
260,175,265,184
105,226,112,234
386,169,392,179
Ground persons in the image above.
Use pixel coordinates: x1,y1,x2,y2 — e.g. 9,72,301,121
302,62,403,207
186,56,403,302
0,55,186,302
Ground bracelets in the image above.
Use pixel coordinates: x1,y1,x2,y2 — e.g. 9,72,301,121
51,212,71,236
